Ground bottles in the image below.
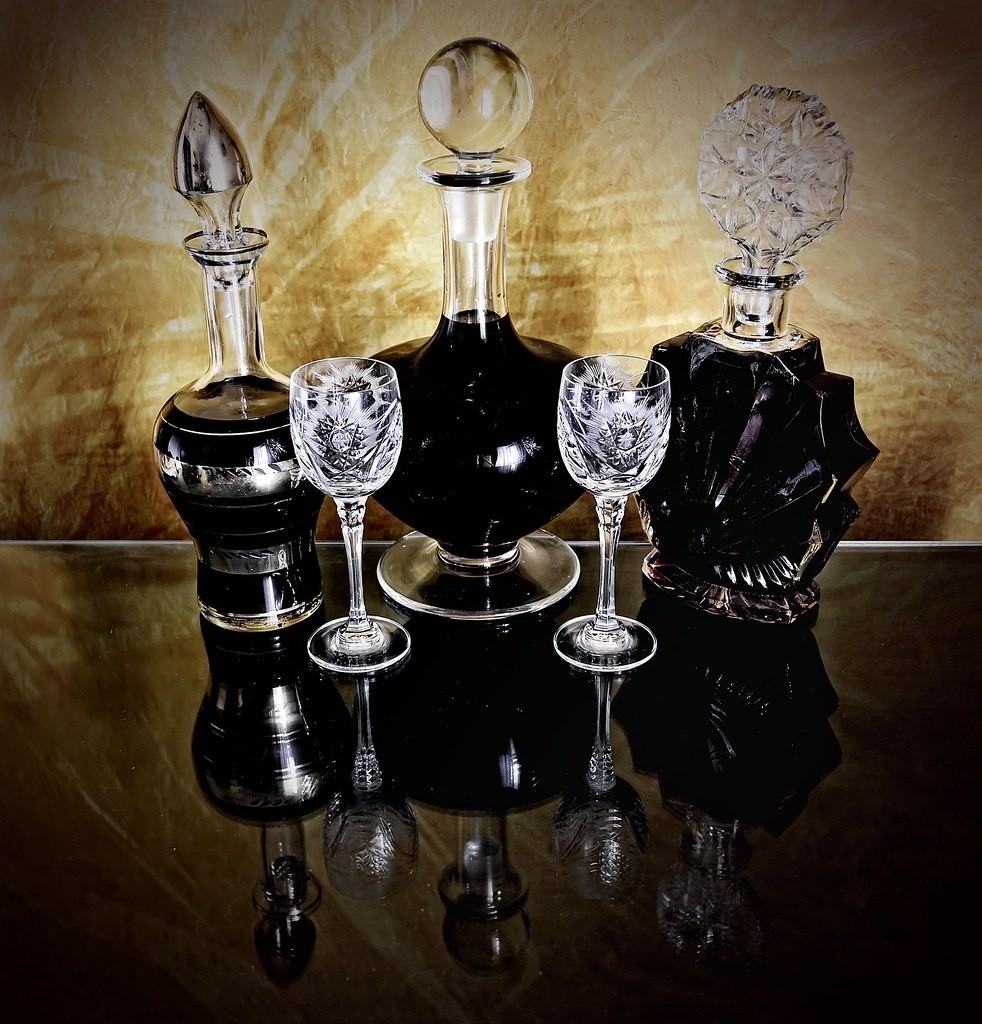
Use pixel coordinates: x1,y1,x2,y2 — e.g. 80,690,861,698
632,84,880,622
338,38,610,622
614,594,841,956
151,90,328,635
377,569,602,978
189,618,353,988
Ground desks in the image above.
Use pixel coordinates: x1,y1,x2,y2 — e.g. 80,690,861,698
1,538,982,1022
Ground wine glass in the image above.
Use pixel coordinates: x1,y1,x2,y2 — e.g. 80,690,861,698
554,352,673,672
322,674,418,904
289,357,412,673
557,666,649,903
